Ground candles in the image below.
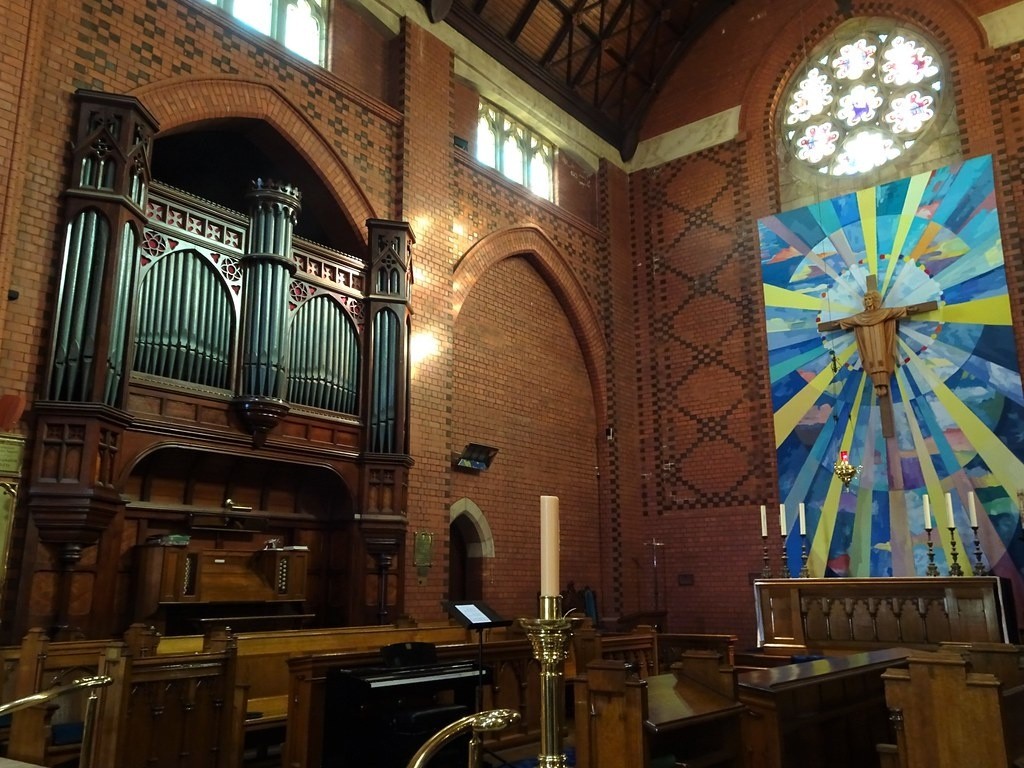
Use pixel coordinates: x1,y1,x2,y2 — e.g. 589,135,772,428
780,504,787,535
945,493,955,528
799,503,807,534
760,505,768,537
923,494,932,529
540,496,561,597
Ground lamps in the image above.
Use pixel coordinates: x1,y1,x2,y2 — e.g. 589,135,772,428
457,442,499,470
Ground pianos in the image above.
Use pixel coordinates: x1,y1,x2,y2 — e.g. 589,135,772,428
322,640,497,768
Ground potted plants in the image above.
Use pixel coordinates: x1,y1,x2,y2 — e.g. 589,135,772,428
969,492,977,527
